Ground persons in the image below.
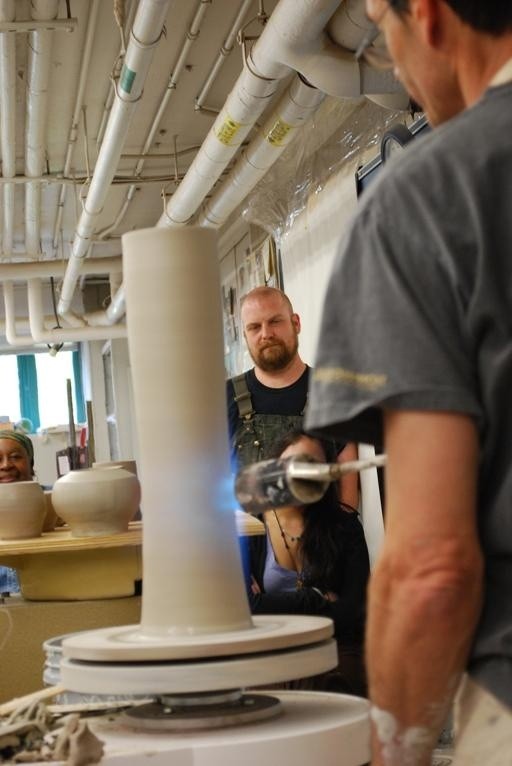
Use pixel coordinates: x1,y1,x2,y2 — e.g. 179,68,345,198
0,430,34,483
241,431,371,699
226,287,357,513
301,0,512,766
